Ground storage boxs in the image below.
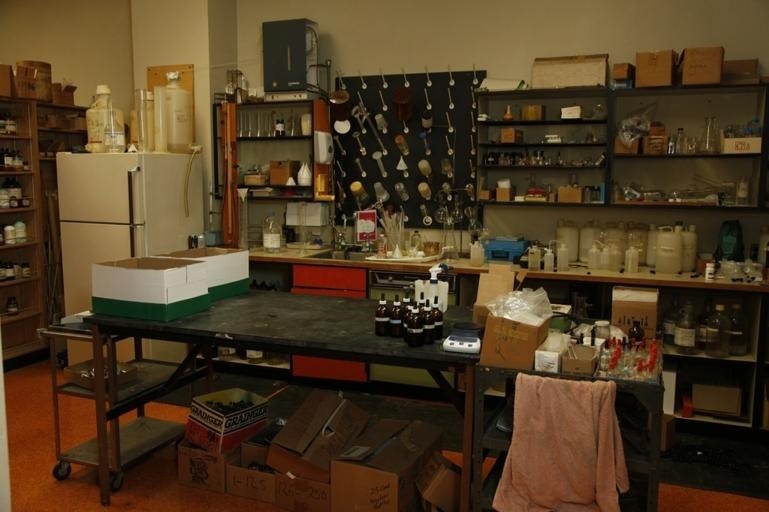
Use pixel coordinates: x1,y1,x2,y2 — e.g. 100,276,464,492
613,284,658,345
239,443,269,468
266,389,370,484
530,55,608,87
17,59,52,99
634,50,676,88
275,468,330,510
183,387,268,457
679,46,725,85
148,443,174,464
0,65,15,98
171,247,253,299
174,447,223,493
479,313,553,372
330,416,444,512
224,456,278,503
416,452,462,511
89,256,212,323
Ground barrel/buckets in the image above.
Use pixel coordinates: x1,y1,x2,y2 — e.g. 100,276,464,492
167,72,194,154
86,86,125,152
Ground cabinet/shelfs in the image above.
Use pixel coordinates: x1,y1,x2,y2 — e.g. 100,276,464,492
220,95,328,249
37,327,211,477
476,86,608,202
0,100,47,369
31,103,89,335
473,367,664,511
609,89,769,206
458,267,768,499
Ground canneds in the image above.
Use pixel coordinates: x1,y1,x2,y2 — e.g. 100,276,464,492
21,262,32,278
22,196,30,207
9,196,18,208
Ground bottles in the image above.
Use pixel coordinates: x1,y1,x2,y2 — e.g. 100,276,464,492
0,145,30,171
0,183,9,208
728,303,749,355
404,303,413,342
471,241,484,266
4,222,16,244
388,295,403,337
557,244,569,273
528,245,540,272
423,299,434,345
628,319,645,349
5,112,16,136
376,234,387,259
595,337,663,386
673,304,698,355
13,216,27,243
401,293,412,315
5,260,15,280
263,210,282,254
8,176,23,209
104,110,126,154
408,306,423,348
706,303,731,357
375,293,390,336
0,114,5,136
625,246,639,273
705,263,715,281
417,292,425,315
433,296,443,340
7,296,18,315
544,249,554,272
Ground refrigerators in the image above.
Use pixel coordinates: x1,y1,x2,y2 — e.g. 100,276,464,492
55,152,204,376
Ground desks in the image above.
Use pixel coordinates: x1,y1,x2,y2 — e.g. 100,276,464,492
91,289,476,512
